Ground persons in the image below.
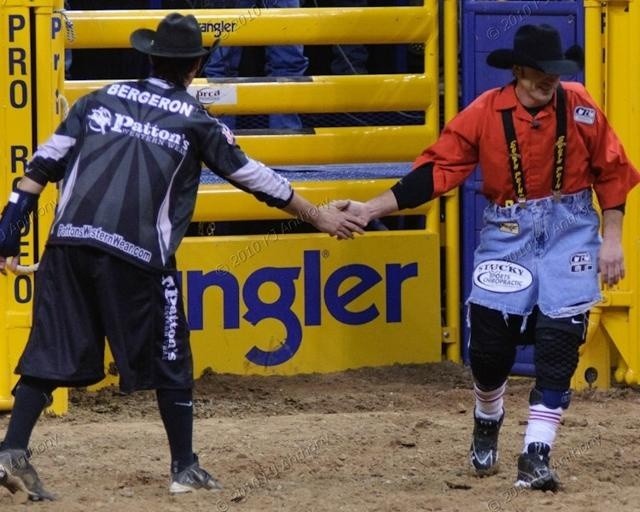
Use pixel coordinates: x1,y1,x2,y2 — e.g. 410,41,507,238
0,12,368,501
330,0,425,125
201,0,309,130
328,24,640,493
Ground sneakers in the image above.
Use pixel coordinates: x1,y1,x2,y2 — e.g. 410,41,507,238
515,441,560,496
467,402,506,478
0,441,59,504
168,452,225,497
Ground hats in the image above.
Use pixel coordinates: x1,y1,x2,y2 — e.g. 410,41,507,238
129,13,221,60
485,21,581,78
